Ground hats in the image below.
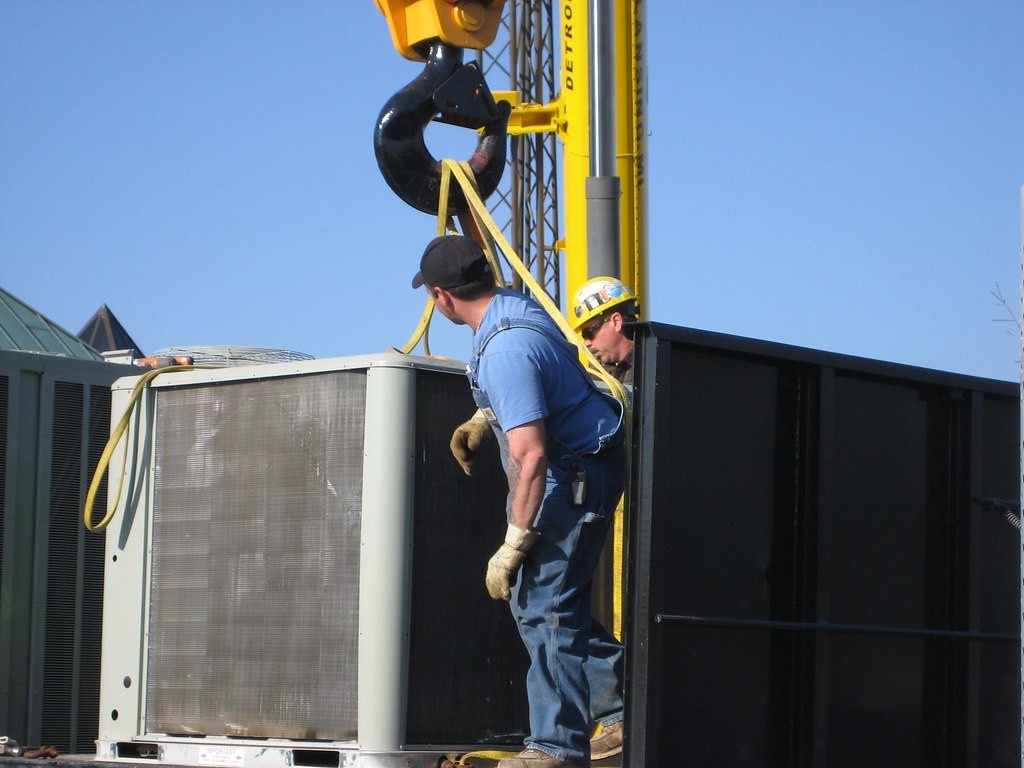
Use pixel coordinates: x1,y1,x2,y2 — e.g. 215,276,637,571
412,235,489,289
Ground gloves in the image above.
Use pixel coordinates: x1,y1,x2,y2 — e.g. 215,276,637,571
450,409,489,476
485,521,542,601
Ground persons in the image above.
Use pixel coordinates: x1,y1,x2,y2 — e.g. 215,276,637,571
412,236,623,768
571,276,640,477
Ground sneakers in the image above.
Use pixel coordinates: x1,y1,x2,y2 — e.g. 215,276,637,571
496,745,569,768
589,719,625,762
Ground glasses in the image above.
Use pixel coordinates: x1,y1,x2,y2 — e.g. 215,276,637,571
582,313,610,338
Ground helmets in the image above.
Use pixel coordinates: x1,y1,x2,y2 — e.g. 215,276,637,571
572,276,639,330
